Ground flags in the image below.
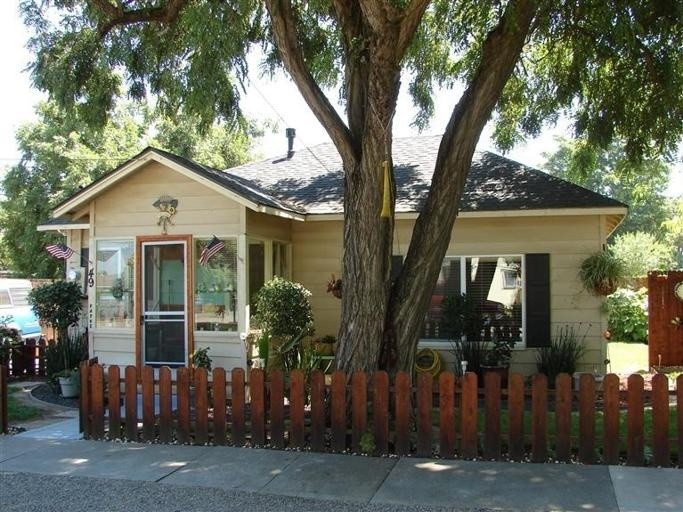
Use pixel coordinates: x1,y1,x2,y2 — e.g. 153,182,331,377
198,236,224,268
45,242,72,260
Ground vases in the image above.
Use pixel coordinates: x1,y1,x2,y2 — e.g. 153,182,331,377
573,370,605,402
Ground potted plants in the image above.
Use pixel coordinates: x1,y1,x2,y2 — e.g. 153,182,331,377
252,279,316,409
46,335,86,398
463,315,517,400
571,251,627,306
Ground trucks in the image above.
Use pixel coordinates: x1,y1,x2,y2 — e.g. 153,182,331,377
0,278,42,347
